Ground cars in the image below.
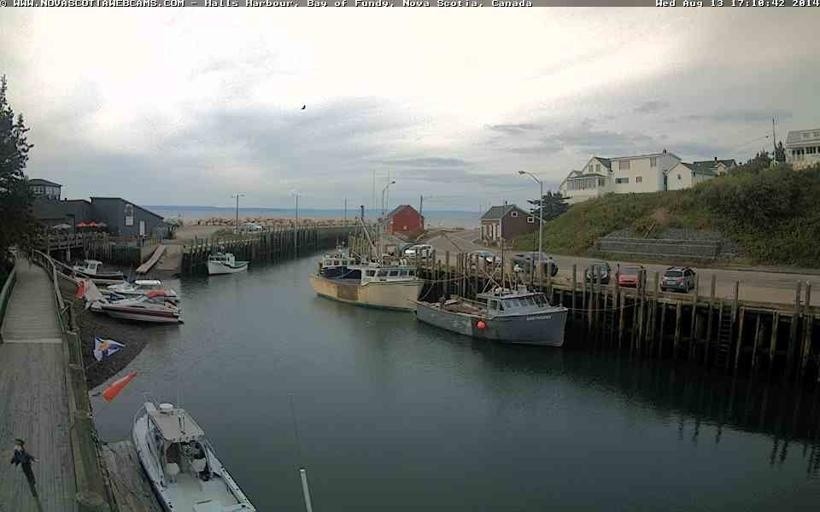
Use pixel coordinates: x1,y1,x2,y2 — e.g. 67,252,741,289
405,245,436,258
584,262,611,284
616,265,648,287
468,251,502,267
239,222,265,231
661,265,696,294
396,243,418,255
512,251,558,277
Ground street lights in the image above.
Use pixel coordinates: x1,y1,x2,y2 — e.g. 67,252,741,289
381,181,395,225
519,170,543,275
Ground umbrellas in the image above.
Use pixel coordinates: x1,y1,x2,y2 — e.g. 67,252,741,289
53,219,108,228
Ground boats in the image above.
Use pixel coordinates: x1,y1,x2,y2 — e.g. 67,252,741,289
68,260,181,325
308,256,423,310
419,259,568,347
132,395,256,512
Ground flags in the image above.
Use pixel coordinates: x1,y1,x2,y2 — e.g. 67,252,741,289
91,335,126,363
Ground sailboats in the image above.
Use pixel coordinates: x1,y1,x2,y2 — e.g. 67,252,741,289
207,253,248,274
